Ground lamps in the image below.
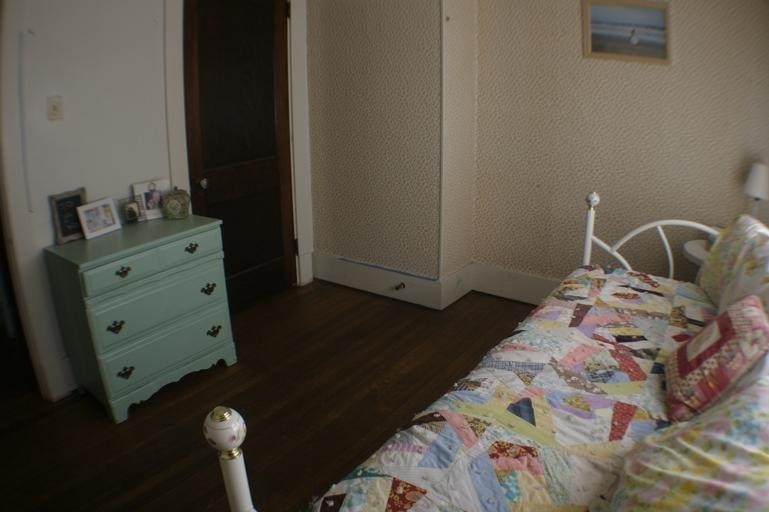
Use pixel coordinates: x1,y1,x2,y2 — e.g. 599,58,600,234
744,164,769,217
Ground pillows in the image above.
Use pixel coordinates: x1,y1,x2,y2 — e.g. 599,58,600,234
664,296,769,420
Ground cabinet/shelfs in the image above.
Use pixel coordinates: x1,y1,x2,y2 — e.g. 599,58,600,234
43,215,236,423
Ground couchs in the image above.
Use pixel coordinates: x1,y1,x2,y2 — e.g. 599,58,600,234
203,190,769,512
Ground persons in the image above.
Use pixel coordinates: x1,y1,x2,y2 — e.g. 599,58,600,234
128,206,137,218
146,191,161,210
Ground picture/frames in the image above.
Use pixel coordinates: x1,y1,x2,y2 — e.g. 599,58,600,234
133,178,171,220
76,196,122,240
48,187,89,245
582,0,673,64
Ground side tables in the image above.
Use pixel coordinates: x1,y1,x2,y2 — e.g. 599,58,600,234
683,240,713,265
